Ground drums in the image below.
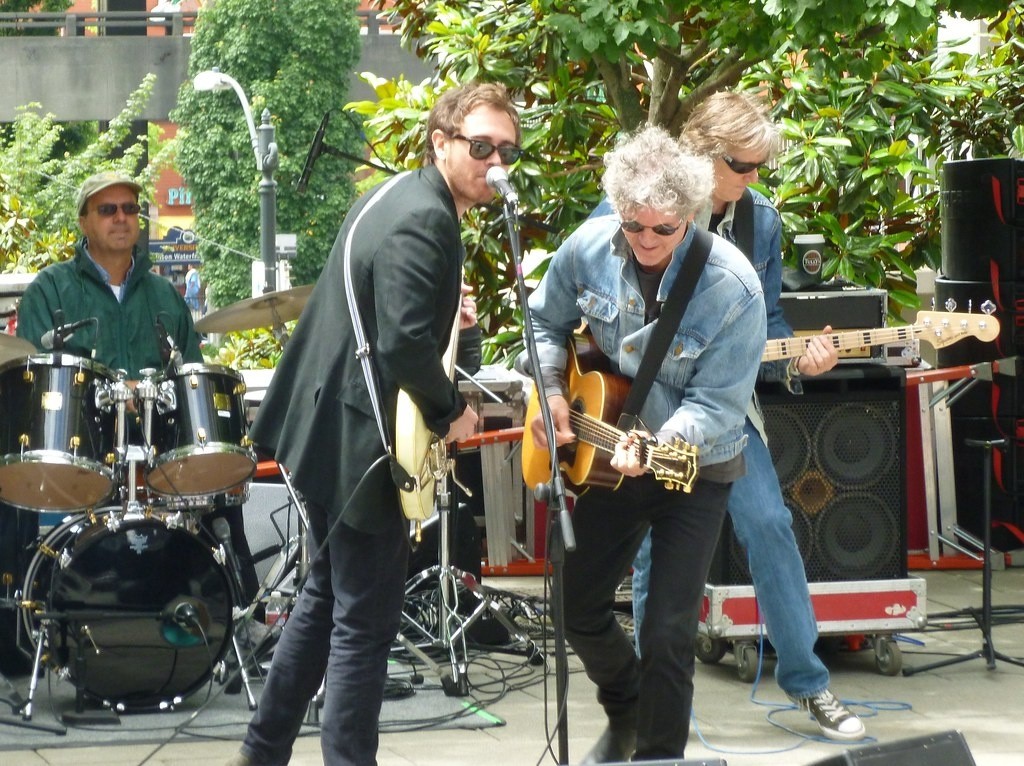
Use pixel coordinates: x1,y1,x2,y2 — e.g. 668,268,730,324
138,358,260,499
14,503,241,723
1,353,123,512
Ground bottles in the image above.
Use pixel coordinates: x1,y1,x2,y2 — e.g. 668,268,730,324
265,591,288,631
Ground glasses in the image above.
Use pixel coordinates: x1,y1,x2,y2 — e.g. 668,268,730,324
617,213,686,235
723,153,769,175
447,131,524,165
87,202,142,217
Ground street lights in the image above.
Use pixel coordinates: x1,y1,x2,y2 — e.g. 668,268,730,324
191,68,280,296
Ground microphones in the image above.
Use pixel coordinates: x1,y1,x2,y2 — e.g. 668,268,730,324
176,603,201,627
155,321,183,368
212,517,243,586
486,165,519,208
40,316,98,349
296,113,330,193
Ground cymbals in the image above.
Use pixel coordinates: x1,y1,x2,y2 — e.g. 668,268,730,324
1,331,38,363
195,281,318,335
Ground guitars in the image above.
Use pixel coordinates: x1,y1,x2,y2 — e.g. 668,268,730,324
521,328,697,501
761,295,1001,364
394,292,465,546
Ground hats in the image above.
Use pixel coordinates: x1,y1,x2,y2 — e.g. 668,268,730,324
77,169,143,217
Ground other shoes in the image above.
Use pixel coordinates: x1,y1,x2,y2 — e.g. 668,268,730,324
236,619,269,644
577,718,638,766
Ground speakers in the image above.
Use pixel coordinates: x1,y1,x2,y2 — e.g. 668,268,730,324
807,730,976,766
932,156,1024,554
708,363,906,585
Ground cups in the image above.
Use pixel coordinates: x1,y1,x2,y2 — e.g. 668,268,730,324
794,233,826,288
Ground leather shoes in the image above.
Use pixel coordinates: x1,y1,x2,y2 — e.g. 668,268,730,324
381,677,416,700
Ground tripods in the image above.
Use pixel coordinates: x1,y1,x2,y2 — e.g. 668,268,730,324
402,440,547,695
902,435,1024,679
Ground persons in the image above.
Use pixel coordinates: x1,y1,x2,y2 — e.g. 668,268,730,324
514,129,768,763
233,83,520,766
18,172,282,662
586,92,866,740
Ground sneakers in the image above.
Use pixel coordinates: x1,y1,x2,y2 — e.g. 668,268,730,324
785,685,867,741
